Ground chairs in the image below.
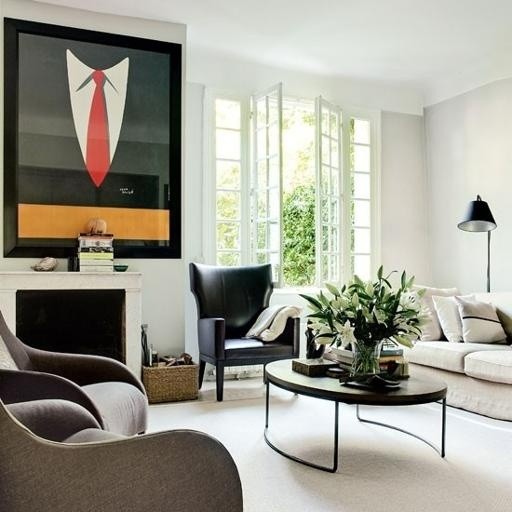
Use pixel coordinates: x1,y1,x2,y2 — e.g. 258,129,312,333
1,307,151,438
188,261,301,403
1,395,244,511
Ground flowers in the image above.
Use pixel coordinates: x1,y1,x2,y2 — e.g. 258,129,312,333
300,262,436,379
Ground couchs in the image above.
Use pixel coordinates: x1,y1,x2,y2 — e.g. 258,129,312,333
381,289,512,422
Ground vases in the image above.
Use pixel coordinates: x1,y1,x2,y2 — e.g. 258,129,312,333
349,339,382,380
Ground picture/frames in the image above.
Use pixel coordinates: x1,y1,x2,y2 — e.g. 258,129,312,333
2,16,183,260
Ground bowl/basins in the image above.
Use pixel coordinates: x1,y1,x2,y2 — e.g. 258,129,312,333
114,264,130,272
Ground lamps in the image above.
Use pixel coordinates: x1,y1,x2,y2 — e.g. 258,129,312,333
457,194,497,293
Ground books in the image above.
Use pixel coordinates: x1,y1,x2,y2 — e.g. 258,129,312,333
71,232,116,272
336,347,410,378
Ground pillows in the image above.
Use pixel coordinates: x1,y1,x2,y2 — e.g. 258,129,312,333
455,295,508,345
403,282,459,343
432,294,483,342
495,306,511,341
